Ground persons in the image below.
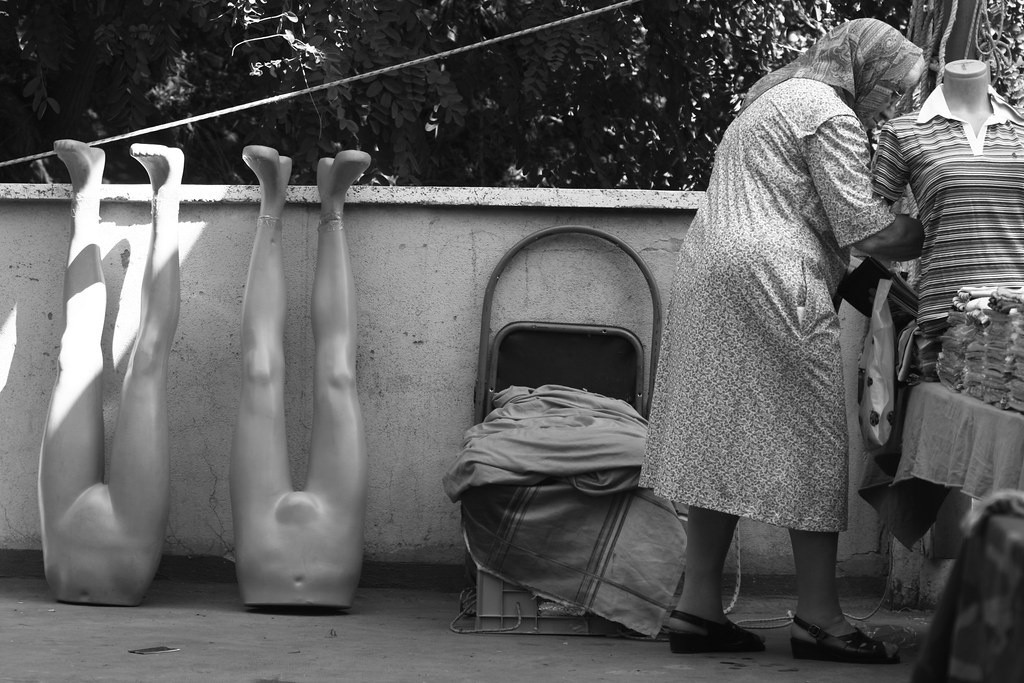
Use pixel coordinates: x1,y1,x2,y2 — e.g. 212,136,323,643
36,138,185,608
635,18,926,664
227,146,373,610
869,60,1024,326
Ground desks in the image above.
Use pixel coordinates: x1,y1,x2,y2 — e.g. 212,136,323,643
888,377,1024,502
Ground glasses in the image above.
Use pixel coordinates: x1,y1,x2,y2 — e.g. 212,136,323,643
896,91,906,107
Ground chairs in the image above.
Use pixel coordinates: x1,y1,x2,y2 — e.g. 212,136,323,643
907,491,1024,683
472,222,664,426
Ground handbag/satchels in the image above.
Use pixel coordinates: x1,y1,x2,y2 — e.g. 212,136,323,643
856,279,896,452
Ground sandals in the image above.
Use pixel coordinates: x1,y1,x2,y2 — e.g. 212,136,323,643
669,610,764,652
790,615,900,665
